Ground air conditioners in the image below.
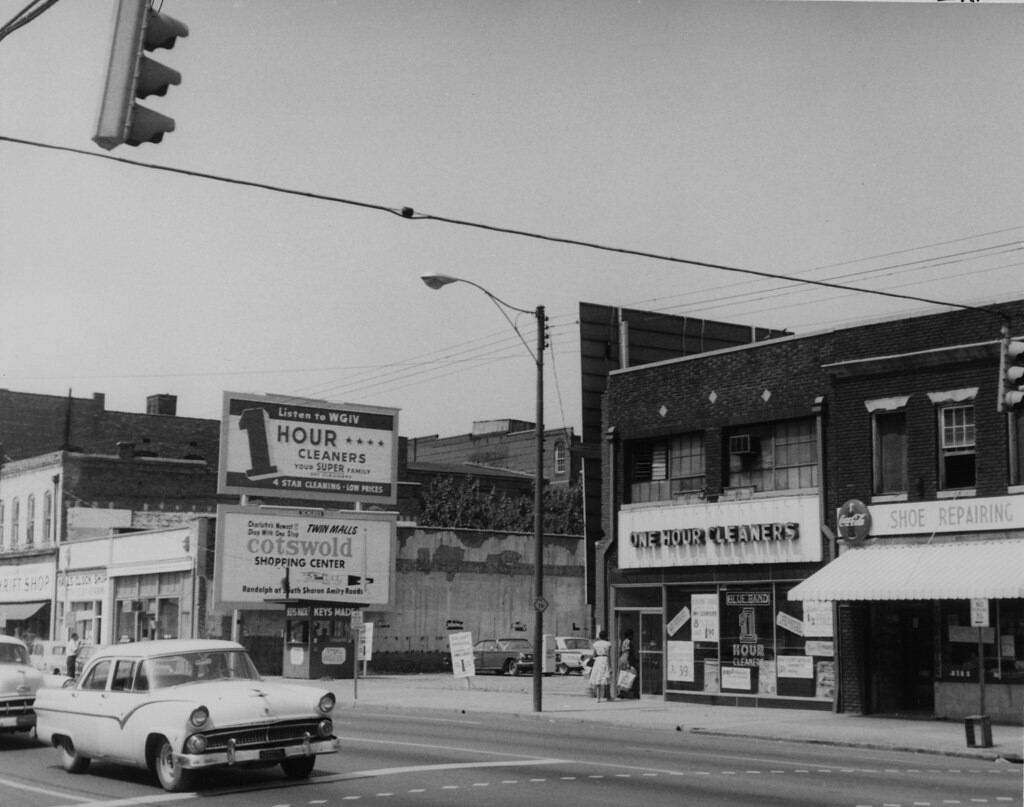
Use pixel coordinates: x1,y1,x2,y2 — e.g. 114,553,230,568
730,434,754,454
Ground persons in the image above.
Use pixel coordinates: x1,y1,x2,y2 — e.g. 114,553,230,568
315,622,330,641
65,633,80,677
588,630,639,702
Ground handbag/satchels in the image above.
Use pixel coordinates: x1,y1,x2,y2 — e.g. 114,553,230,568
617,667,636,689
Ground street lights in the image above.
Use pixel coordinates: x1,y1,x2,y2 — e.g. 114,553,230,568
421,274,550,713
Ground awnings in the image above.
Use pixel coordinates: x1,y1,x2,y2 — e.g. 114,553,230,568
786,538,1024,600
0,603,47,620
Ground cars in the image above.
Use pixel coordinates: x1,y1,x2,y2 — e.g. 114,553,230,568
443,637,534,677
25,638,177,698
35,639,341,794
544,636,599,676
0,634,78,734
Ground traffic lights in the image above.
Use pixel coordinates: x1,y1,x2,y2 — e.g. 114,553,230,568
92,1,191,151
997,337,1024,415
